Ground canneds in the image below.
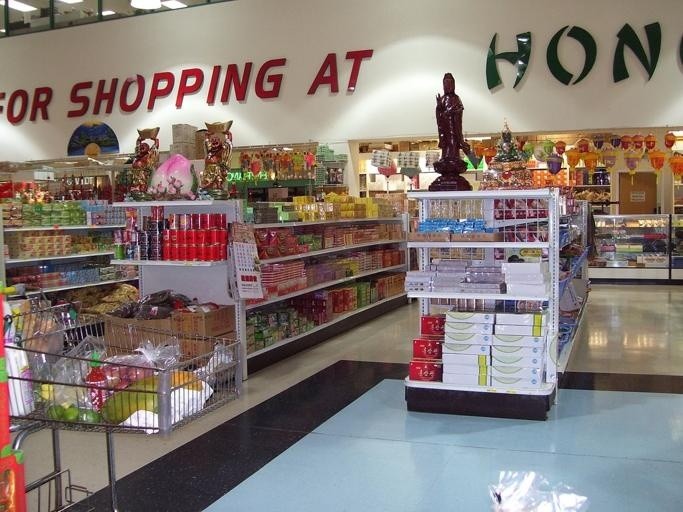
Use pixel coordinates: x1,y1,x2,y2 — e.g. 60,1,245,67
114,206,229,262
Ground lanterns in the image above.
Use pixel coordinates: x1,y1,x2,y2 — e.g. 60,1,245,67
376,132,682,189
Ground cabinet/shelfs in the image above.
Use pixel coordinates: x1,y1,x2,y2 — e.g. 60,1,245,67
1,159,410,419
406,161,681,421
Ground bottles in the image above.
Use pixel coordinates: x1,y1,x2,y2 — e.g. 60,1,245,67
84,350,109,420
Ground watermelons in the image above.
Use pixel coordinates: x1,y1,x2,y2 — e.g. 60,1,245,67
102,370,202,425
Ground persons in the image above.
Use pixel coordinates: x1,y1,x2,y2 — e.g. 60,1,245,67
434,73,464,160
200,131,231,191
127,132,161,192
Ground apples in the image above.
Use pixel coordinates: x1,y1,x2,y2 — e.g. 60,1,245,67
48,405,64,418
86,411,100,422
63,407,80,421
